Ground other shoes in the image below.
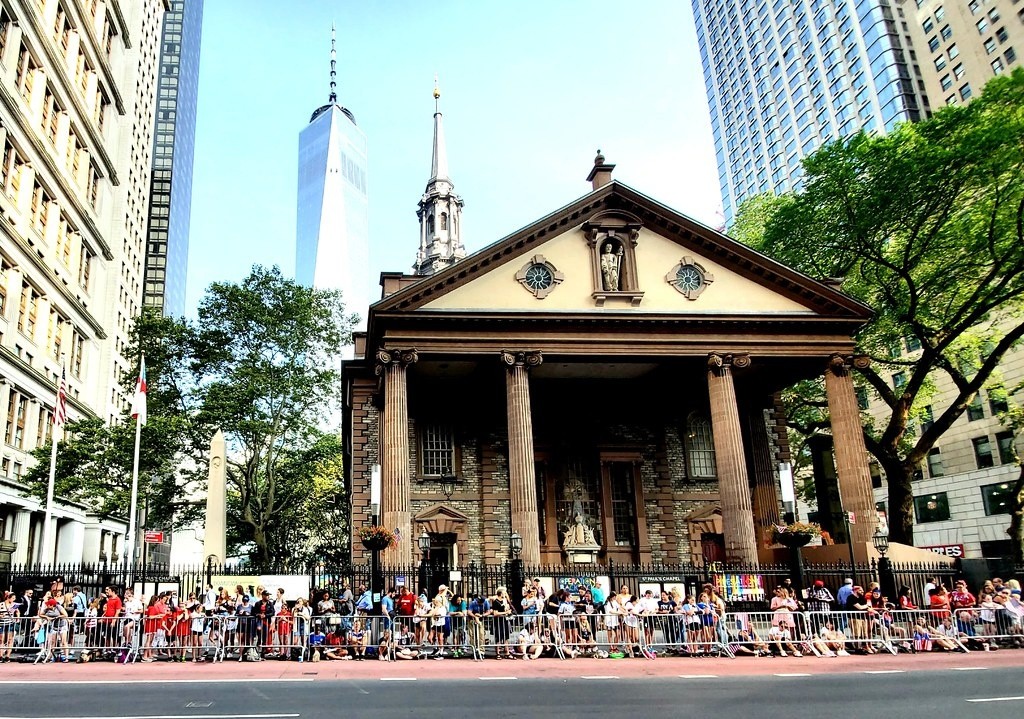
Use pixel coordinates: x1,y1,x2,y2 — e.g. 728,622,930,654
0,656,3,663
838,649,849,657
855,640,999,655
823,650,837,658
780,651,788,657
793,651,803,657
35,642,689,671
4,657,10,663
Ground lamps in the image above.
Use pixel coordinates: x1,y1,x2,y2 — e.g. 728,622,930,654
871,526,889,559
438,467,458,503
509,530,524,560
418,526,431,559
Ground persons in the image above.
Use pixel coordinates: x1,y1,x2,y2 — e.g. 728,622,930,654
600,243,620,291
0,576,1024,664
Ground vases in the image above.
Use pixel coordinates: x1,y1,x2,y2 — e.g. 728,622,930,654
361,536,391,551
775,532,812,547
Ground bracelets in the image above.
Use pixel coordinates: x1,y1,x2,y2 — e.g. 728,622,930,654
54,617,56,620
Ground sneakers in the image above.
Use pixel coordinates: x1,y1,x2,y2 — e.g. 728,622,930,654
766,652,775,658
757,651,765,656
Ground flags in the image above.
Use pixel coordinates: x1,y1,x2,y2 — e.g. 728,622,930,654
52,365,66,442
130,354,148,426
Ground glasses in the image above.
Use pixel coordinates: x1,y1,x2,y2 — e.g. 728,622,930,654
190,598,195,599
1002,591,1008,595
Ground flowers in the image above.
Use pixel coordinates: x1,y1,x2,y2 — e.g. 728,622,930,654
355,524,398,556
766,522,822,546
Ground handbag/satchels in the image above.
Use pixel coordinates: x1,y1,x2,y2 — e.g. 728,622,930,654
338,601,351,616
13,621,20,631
329,611,342,625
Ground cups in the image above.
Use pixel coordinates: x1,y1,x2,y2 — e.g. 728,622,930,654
299,656,303,663
114,657,118,663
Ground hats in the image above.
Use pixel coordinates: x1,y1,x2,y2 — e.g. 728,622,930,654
815,580,824,587
476,596,485,603
242,595,250,601
261,590,272,595
45,599,56,606
845,578,852,584
439,584,449,591
956,580,966,588
